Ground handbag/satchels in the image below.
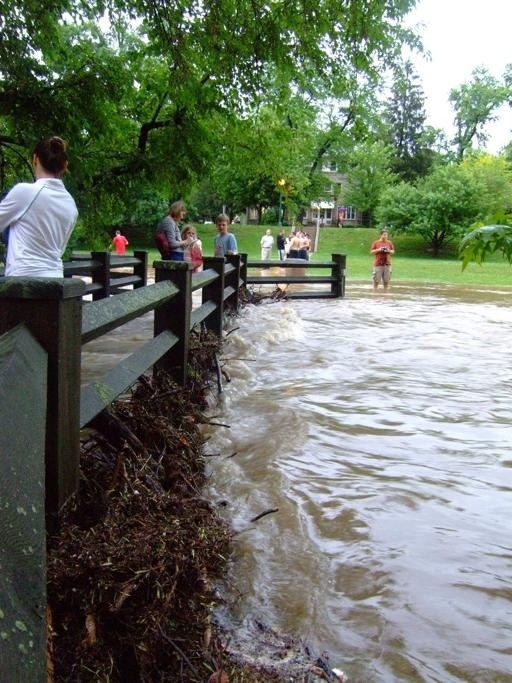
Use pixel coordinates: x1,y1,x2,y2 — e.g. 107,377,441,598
155,230,172,258
185,242,203,270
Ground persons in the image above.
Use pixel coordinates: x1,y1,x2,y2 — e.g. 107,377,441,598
181,225,203,273
0,135,79,278
214,213,238,263
276,228,312,269
259,228,275,270
232,214,240,223
337,211,344,227
370,229,394,289
157,201,194,261
109,231,129,255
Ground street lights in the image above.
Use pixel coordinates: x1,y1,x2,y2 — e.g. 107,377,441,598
277,178,285,226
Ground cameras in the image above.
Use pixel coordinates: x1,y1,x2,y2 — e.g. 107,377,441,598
382,247,387,254
